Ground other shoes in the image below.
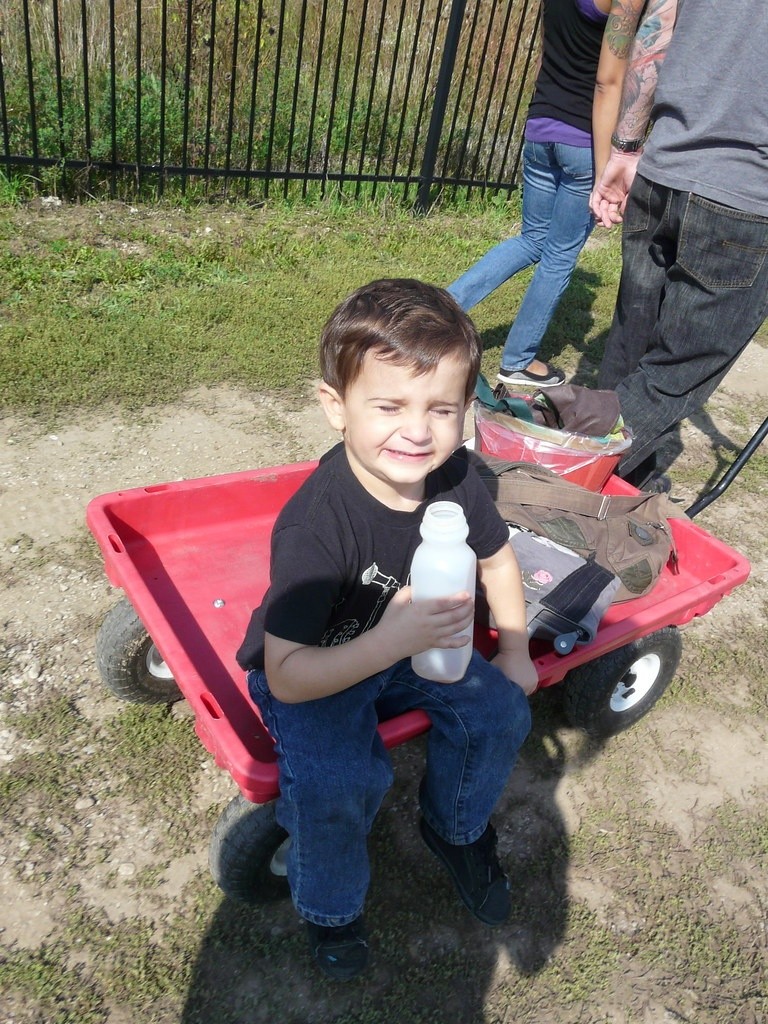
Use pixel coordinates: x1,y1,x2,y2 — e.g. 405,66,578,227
496,362,566,387
648,473,671,495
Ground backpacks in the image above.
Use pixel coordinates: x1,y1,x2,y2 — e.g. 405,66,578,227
457,446,680,605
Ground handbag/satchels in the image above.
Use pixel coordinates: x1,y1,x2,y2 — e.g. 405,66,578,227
476,520,621,655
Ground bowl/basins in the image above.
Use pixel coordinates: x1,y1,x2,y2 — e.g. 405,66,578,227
476,409,630,496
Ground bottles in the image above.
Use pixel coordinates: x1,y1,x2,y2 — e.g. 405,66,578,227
409,501,477,684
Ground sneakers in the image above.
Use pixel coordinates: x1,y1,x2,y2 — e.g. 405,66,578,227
419,816,512,925
299,914,368,986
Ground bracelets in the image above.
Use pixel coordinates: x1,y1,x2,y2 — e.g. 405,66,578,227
611,131,645,153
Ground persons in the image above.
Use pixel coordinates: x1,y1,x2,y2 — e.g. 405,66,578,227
587,0,767,491
236,278,540,979
439,0,647,388
